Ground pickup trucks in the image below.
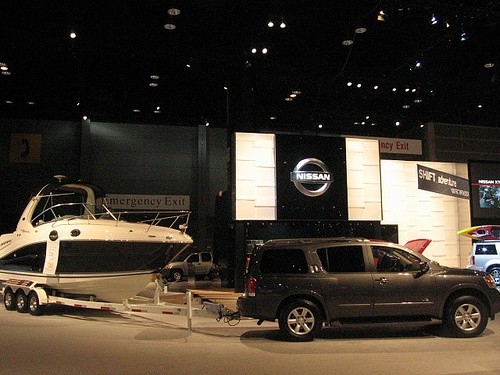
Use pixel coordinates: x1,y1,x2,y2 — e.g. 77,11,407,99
164,252,219,282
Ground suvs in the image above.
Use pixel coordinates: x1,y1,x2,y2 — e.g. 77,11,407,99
467,240,500,286
236,237,500,342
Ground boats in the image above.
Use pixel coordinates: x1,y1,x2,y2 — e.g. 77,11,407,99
0,174,193,303
